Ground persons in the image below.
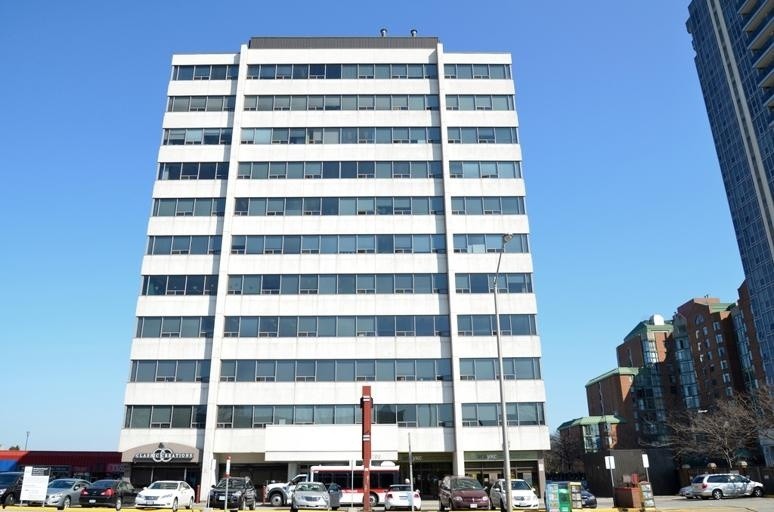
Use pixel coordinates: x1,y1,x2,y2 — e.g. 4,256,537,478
328,480,342,510
405,478,412,492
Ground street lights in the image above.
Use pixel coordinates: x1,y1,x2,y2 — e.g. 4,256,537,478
488,233,519,510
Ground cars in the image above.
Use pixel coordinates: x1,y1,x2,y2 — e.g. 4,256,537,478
382,484,422,511
27,476,91,510
290,480,331,512
79,479,139,511
550,481,596,509
678,483,702,500
546,478,554,484
133,478,196,511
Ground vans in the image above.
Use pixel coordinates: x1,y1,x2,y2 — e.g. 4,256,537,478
692,473,765,500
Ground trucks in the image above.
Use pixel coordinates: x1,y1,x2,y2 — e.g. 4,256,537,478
265,465,399,507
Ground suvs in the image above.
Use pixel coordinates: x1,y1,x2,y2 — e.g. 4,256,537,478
437,476,492,511
487,479,540,511
206,475,256,511
0,472,23,506
